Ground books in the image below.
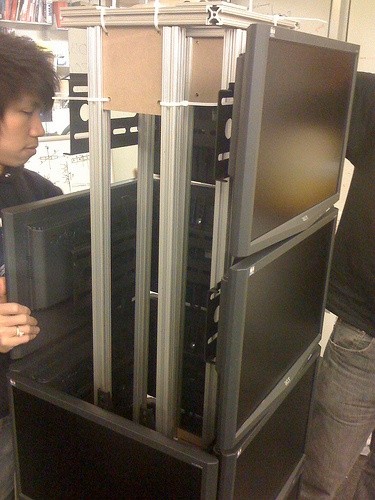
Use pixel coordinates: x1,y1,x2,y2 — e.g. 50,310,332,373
0,0,80,29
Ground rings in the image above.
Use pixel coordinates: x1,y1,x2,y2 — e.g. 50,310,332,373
16,325,24,336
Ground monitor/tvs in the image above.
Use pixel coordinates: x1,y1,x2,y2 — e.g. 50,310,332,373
0,22,362,500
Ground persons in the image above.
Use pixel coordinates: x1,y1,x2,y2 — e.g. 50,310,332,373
300,69,375,500
0,30,65,500
